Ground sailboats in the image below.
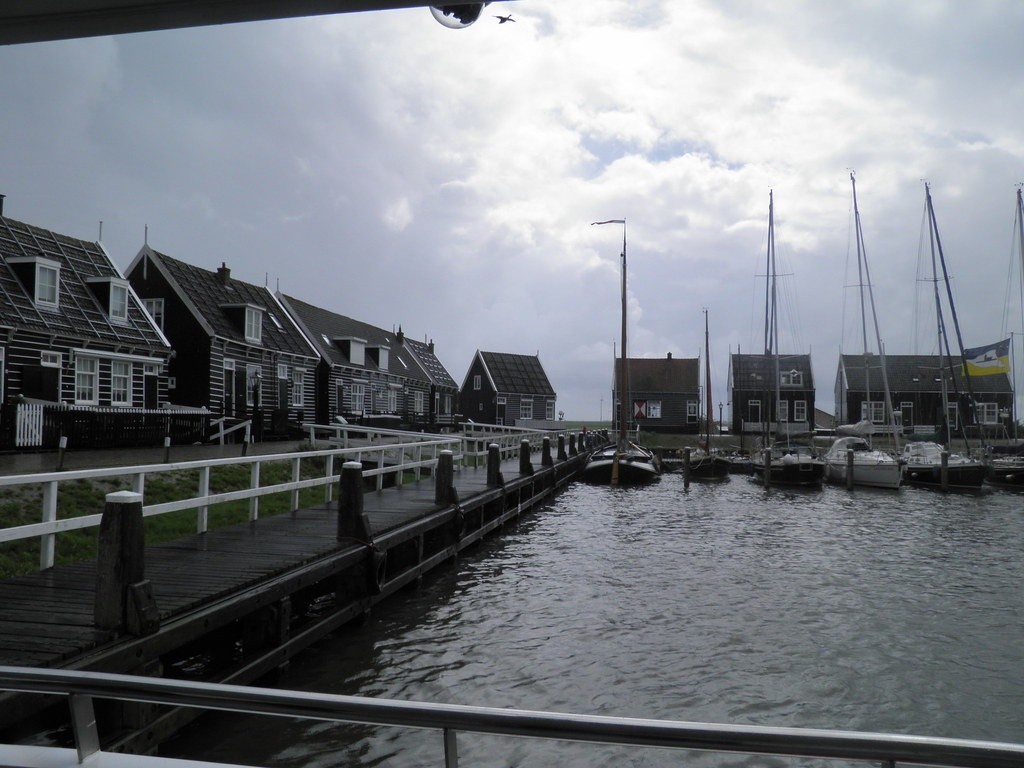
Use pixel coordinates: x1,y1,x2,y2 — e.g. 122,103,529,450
680,308,734,482
821,165,911,491
878,181,998,493
721,187,830,490
578,220,664,487
973,180,1024,491
